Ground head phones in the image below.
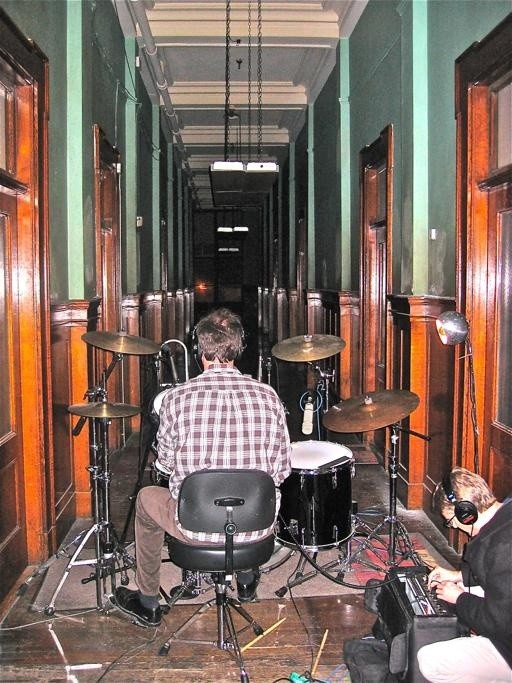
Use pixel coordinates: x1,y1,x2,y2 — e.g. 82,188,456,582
190,319,248,354
442,466,478,524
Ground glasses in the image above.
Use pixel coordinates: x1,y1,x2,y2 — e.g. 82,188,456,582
443,514,455,529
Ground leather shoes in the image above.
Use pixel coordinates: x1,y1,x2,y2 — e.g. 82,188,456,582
236,567,260,603
114,586,161,627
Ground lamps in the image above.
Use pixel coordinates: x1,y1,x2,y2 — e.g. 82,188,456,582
205,2,280,210
435,310,479,472
215,210,251,236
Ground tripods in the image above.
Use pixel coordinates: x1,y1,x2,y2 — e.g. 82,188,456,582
336,429,422,584
275,513,390,597
16,418,171,615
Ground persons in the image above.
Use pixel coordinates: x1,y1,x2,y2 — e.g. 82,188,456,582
110,308,293,628
427,465,512,669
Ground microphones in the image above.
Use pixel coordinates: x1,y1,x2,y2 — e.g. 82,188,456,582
300,401,315,435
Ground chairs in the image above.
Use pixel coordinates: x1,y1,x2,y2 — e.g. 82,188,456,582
158,469,282,683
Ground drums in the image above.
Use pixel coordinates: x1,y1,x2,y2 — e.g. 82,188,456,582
274,441,355,553
151,388,174,425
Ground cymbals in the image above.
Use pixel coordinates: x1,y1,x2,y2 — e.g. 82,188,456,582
271,334,347,363
82,332,161,355
69,401,145,419
323,390,419,433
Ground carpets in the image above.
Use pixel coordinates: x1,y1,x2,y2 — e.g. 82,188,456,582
28,531,444,614
